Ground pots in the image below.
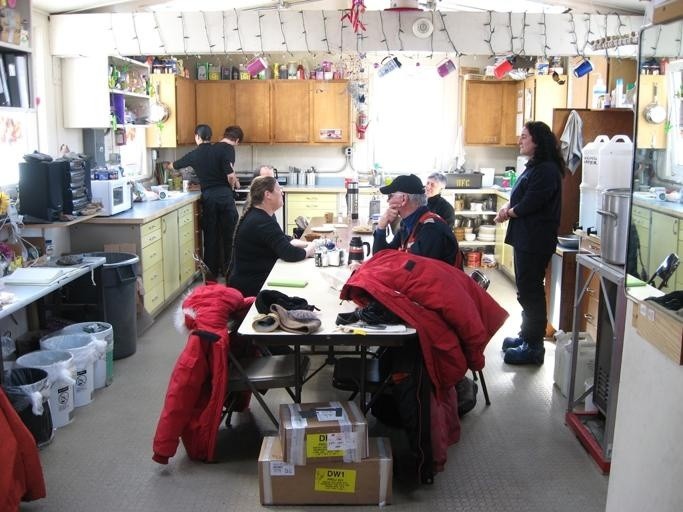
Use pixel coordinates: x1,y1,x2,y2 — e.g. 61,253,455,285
595,188,630,268
642,83,665,125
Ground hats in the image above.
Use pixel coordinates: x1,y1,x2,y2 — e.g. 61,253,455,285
270,304,320,334
380,174,425,194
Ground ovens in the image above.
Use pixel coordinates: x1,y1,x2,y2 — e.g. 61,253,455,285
234,201,284,233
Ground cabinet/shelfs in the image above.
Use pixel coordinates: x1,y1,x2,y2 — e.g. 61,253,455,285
283,192,336,238
635,73,666,150
515,72,567,148
177,201,195,285
146,73,197,149
458,78,516,148
268,78,350,146
572,228,600,344
446,194,512,268
161,209,179,302
628,203,682,295
0,0,32,107
71,218,163,315
193,77,269,145
61,53,153,128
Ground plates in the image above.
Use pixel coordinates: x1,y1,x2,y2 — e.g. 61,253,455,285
310,226,336,233
476,225,495,242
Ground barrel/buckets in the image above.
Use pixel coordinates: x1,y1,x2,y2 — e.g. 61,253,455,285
222,64,233,80
208,63,222,80
196,58,209,81
238,64,251,81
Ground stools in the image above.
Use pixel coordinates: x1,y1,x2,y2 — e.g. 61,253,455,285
330,356,381,402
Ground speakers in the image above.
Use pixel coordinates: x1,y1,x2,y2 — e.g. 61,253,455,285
20,160,64,221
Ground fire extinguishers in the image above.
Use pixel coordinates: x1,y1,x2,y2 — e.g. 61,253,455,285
356,110,369,139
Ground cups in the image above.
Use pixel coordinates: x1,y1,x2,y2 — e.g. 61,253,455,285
159,190,168,200
434,57,456,78
289,172,315,185
325,213,332,223
376,56,400,78
573,60,594,76
494,60,514,79
182,179,190,193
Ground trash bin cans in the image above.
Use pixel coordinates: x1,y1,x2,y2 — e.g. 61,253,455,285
66,251,140,360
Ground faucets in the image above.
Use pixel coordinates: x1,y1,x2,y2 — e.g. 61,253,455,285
453,156,459,172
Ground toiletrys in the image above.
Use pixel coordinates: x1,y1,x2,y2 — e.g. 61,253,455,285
322,61,333,80
315,63,324,80
296,59,305,80
591,74,607,112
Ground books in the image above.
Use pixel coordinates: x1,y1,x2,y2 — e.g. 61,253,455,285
4,265,76,285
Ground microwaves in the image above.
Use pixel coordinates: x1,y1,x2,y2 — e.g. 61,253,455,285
91,177,131,217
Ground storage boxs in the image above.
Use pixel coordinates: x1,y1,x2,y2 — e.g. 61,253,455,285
278,400,370,464
256,435,395,508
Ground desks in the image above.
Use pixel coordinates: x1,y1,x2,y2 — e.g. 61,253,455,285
237,214,428,405
1,252,114,334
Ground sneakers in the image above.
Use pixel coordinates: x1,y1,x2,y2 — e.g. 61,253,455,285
505,343,544,364
503,336,522,352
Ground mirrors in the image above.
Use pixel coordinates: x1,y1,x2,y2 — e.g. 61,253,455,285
624,21,683,322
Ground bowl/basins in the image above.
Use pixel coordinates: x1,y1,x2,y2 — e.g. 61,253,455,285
557,234,578,248
455,227,475,241
304,234,321,242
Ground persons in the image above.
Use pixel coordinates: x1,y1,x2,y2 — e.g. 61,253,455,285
227,176,317,299
371,173,478,417
492,120,567,368
425,173,461,234
242,166,278,222
166,122,239,282
217,127,252,188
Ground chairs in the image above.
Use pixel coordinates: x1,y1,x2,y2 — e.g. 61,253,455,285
217,336,317,431
644,252,679,290
462,269,489,407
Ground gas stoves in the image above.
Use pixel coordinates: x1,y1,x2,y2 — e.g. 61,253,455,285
235,176,286,200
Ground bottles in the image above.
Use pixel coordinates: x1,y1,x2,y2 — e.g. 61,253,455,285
344,181,359,218
321,250,329,267
108,63,150,92
272,60,340,79
346,237,372,264
592,77,624,109
44,239,56,263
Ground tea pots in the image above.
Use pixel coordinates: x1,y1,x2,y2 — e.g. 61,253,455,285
367,169,381,185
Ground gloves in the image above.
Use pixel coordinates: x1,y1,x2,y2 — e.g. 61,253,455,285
252,312,279,333
